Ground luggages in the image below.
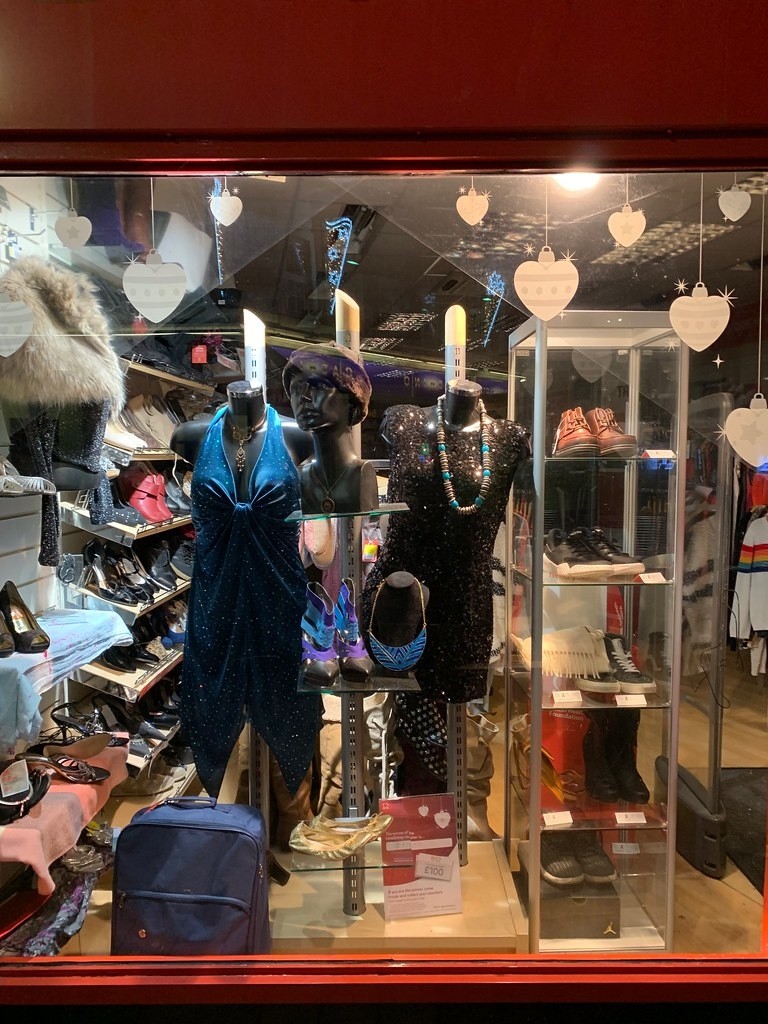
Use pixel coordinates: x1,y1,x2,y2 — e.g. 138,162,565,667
110,796,272,957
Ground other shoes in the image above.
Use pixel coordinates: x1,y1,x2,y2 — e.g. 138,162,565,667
87,275,209,382
89,679,183,757
288,820,373,862
113,742,195,797
81,530,197,606
101,393,188,453
88,462,192,528
308,814,394,841
86,496,194,527
96,599,187,674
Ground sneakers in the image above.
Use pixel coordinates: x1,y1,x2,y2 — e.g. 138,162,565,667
543,527,615,581
604,632,658,694
541,836,585,888
571,528,645,576
570,626,620,694
564,832,619,883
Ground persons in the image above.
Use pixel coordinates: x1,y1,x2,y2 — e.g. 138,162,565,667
361,380,530,703
283,340,379,515
170,380,324,799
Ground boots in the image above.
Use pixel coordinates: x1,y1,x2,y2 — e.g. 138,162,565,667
336,576,375,683
581,709,620,803
300,582,340,687
608,707,650,805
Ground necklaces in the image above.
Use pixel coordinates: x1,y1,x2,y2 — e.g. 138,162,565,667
437,394,491,514
228,406,267,471
312,457,357,513
368,578,426,671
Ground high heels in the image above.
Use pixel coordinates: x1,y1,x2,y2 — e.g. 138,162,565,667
49,705,115,738
1,606,15,659
1,580,50,652
80,819,113,849
16,751,110,788
0,578,49,654
60,845,105,874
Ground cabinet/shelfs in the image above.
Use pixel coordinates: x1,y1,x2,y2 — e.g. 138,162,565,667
62,362,214,805
0,459,133,941
281,500,422,875
503,309,689,955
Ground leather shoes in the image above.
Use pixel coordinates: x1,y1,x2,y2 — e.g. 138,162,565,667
551,407,599,458
583,408,639,458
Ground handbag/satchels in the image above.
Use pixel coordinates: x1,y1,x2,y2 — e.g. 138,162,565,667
123,339,206,382
204,349,245,382
143,287,228,357
